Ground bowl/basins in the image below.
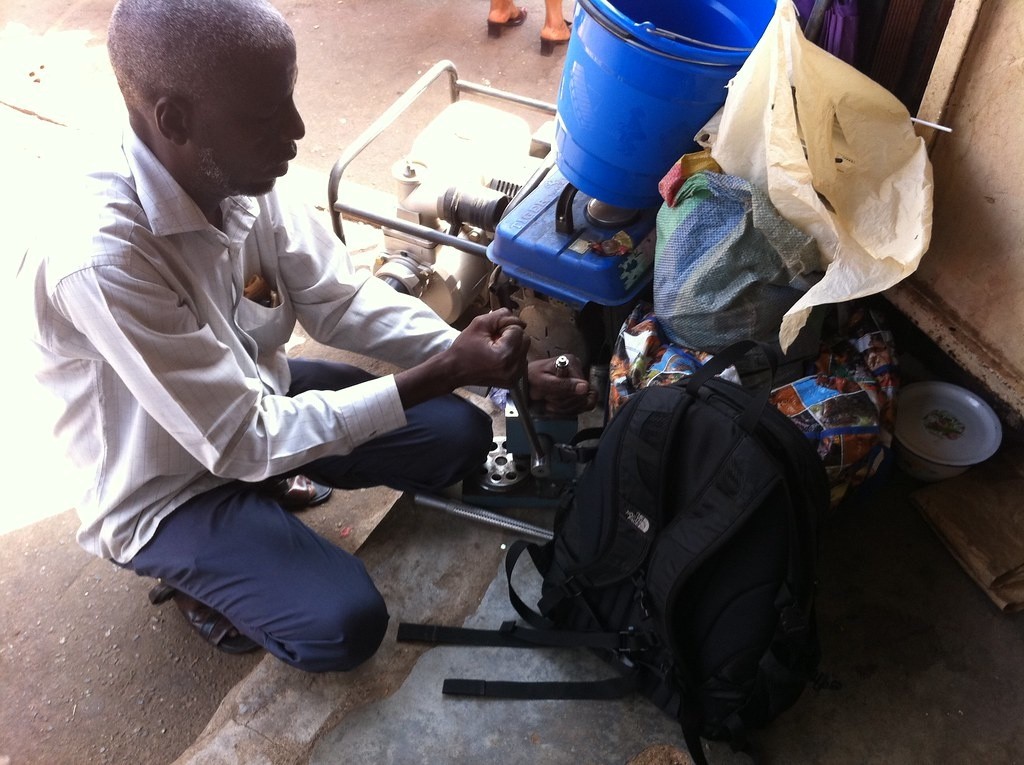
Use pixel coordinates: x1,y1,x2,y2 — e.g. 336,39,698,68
893,437,969,483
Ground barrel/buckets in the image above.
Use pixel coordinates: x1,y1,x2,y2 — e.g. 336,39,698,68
554,0,779,212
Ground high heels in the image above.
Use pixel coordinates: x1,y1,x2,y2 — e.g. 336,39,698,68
487,6,528,39
540,20,573,55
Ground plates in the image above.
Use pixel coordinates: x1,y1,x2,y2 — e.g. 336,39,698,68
891,380,1002,467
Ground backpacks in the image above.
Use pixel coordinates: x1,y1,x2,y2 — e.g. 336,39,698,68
504,339,832,749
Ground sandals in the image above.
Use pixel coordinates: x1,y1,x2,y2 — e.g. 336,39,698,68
258,472,331,508
147,580,262,653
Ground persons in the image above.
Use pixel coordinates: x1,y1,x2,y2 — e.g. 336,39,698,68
486,0,572,56
12,0,599,674
